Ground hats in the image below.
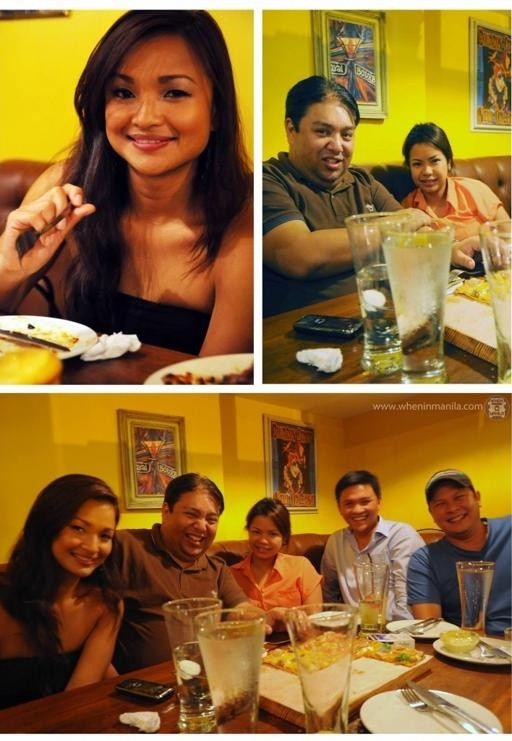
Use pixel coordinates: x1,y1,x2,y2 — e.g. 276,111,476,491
425,468,476,499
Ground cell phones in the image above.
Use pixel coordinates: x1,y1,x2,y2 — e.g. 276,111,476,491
117,678,173,704
294,314,363,340
450,256,485,277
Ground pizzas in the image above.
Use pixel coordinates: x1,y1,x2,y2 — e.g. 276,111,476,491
259,630,424,677
453,271,512,308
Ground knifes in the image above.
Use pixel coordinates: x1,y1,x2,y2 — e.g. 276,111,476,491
479,639,511,660
2,326,71,349
405,679,495,733
393,617,439,634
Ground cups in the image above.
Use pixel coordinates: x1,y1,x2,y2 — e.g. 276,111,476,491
161,597,223,733
283,603,361,734
478,219,511,382
344,207,456,384
352,558,392,638
455,560,496,635
195,610,266,734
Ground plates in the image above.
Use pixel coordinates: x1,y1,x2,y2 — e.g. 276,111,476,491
1,314,101,369
140,353,254,384
431,636,512,666
308,610,363,629
361,690,505,733
385,619,461,640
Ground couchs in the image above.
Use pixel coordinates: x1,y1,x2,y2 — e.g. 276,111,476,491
351,155,510,224
2,157,79,318
201,528,446,579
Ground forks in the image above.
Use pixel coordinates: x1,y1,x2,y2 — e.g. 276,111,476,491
398,686,478,733
16,203,73,268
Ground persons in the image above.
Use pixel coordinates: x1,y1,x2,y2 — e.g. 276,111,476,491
80,473,312,681
405,467,511,637
0,10,253,357
321,470,427,623
397,120,512,243
0,473,125,714
262,74,512,318
227,497,324,617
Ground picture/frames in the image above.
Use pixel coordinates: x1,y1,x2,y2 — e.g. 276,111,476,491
116,406,187,515
263,412,319,513
468,15,512,131
312,8,389,120
